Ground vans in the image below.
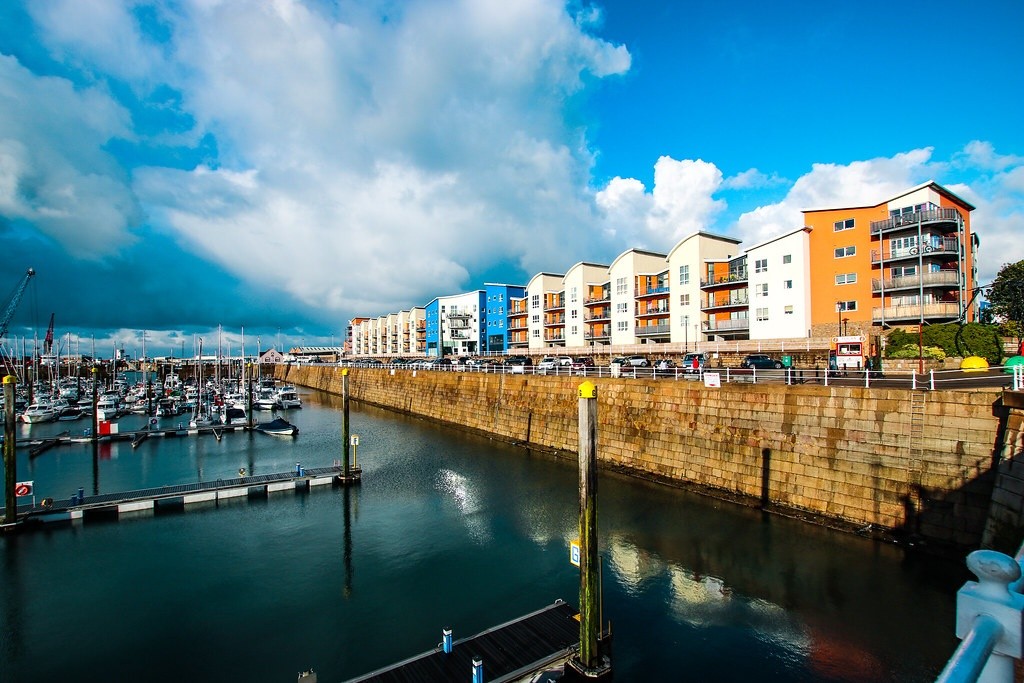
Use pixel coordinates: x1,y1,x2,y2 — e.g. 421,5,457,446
681,352,711,375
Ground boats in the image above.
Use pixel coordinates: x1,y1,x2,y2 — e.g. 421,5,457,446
0,320,302,437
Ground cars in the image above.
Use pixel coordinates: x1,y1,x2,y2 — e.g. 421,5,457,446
571,357,595,371
503,355,532,366
555,355,573,369
652,359,677,373
609,357,631,372
625,355,648,367
309,356,501,366
741,354,782,369
538,357,558,370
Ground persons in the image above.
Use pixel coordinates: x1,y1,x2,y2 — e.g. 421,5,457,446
862,356,872,380
692,355,701,375
660,359,668,379
870,352,885,379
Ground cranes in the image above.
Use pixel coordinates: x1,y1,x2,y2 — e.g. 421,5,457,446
0,267,35,340
44,313,55,355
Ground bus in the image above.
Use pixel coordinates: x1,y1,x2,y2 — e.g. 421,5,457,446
297,356,309,363
